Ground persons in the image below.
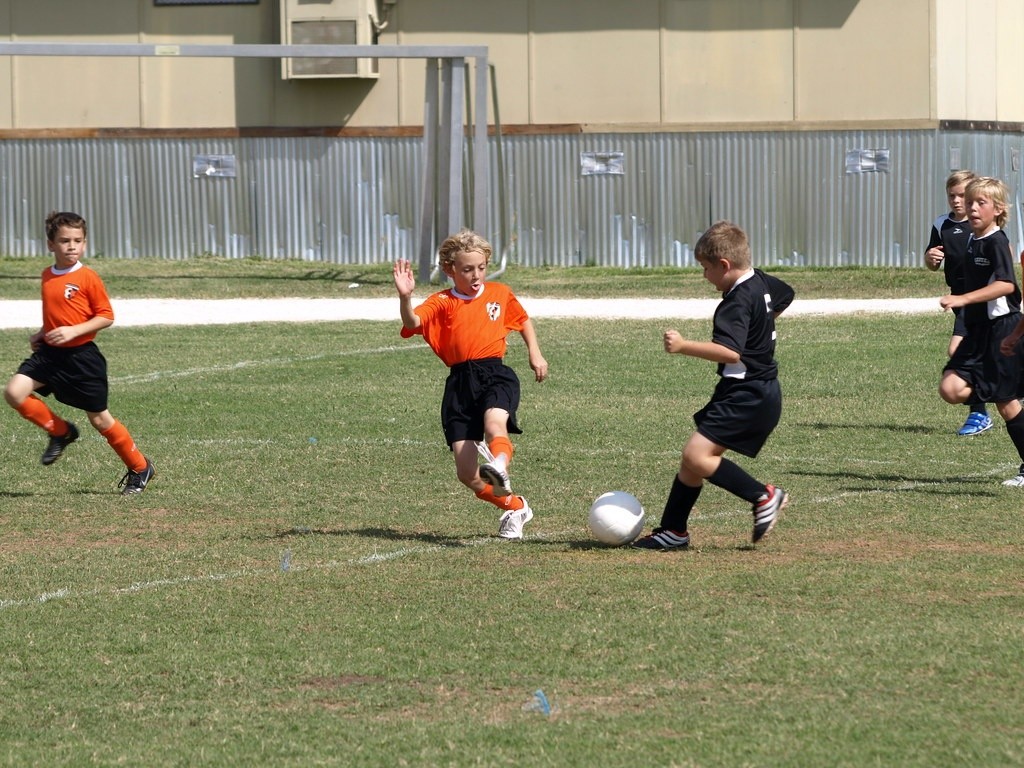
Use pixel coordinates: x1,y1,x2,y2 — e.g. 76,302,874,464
394,231,548,538
5,212,155,493
632,221,794,551
925,171,1024,488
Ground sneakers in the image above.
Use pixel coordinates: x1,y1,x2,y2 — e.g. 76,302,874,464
959,409,994,437
42,420,79,466
750,484,790,545
118,456,157,496
1001,472,1024,487
473,441,513,497
631,528,690,551
497,496,534,541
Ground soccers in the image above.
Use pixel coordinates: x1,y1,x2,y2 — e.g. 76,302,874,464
588,491,645,546
